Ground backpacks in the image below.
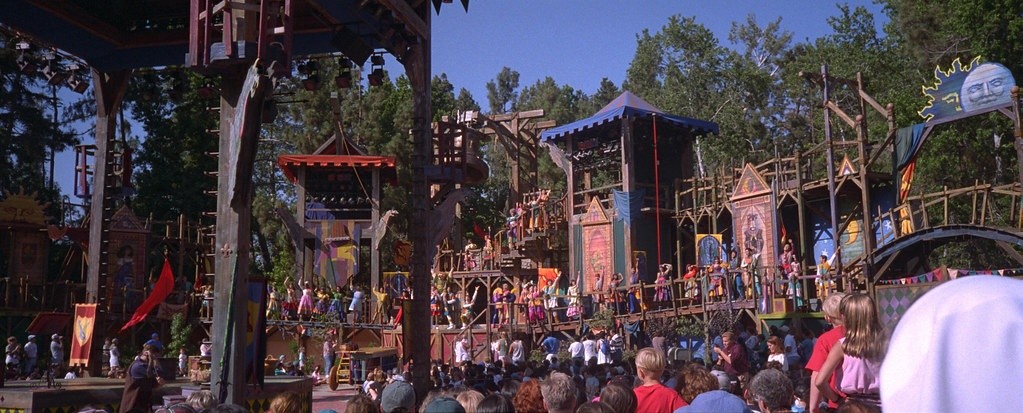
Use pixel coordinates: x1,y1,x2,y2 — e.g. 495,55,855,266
506,293,516,301
599,339,610,354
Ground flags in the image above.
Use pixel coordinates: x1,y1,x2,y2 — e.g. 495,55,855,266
70,305,97,364
117,261,174,333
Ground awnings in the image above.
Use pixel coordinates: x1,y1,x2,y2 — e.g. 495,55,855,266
541,90,718,142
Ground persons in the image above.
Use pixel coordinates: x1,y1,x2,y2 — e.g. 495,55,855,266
264,239,884,413
4,333,248,413
463,188,549,268
199,285,213,318
878,273,1023,413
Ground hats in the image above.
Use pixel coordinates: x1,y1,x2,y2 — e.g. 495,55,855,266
151,333,158,338
28,335,35,340
381,381,416,413
710,370,731,389
275,362,283,367
112,338,117,342
423,397,466,413
779,325,790,332
52,334,58,339
673,390,752,413
284,361,293,367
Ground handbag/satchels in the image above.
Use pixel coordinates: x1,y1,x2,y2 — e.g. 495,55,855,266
708,267,713,272
